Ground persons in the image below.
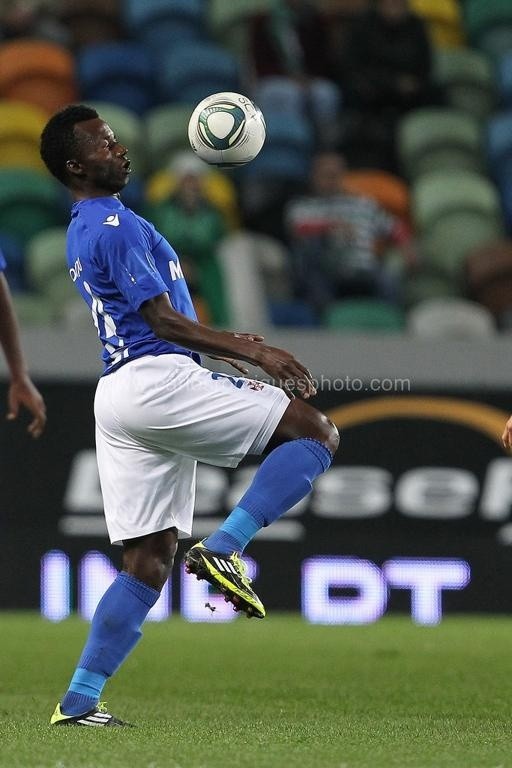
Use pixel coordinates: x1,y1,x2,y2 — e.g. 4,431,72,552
35,103,344,730
501,413,512,449
1,0,511,342
0,253,49,439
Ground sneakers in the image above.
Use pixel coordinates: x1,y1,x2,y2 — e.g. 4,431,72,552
49,701,140,736
183,535,267,620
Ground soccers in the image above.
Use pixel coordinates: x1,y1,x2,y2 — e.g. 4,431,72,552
189,91,268,169
